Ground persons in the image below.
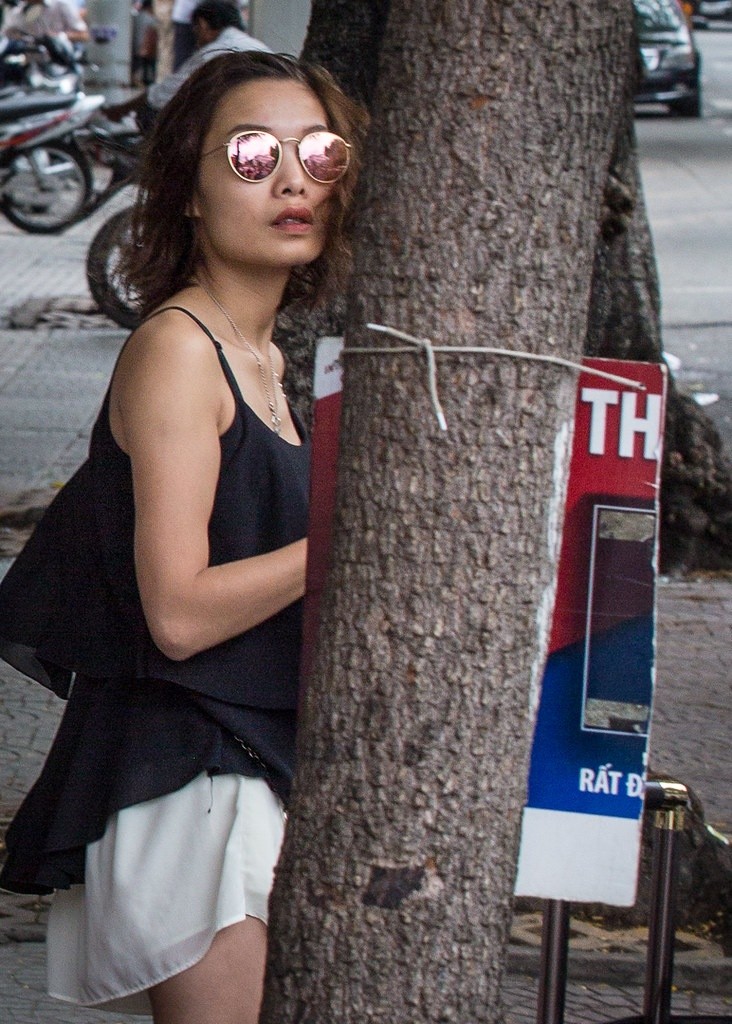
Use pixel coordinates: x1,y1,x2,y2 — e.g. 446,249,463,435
0,0,279,131
0,48,371,1023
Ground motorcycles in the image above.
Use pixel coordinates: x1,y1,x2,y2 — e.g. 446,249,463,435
0,0,165,330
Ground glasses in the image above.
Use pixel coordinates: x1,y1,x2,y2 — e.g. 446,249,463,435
178,131,354,183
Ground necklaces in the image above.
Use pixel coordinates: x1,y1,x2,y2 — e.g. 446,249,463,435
192,273,286,438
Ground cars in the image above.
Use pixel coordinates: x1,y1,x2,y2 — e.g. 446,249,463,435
634,0,701,118
